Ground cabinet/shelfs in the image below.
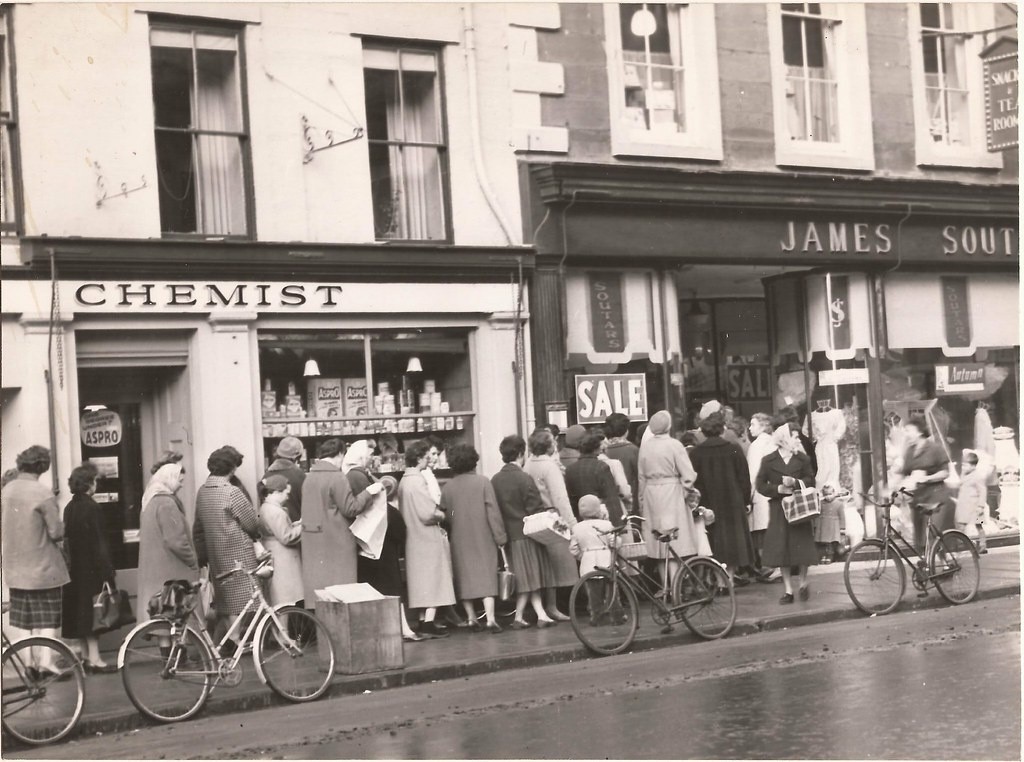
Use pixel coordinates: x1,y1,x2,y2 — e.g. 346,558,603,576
266,427,466,480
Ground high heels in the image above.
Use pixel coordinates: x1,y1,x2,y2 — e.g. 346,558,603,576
37,665,74,683
486,622,502,634
89,664,118,674
80,657,91,675
226,638,252,658
469,620,483,632
25,666,40,682
402,634,424,641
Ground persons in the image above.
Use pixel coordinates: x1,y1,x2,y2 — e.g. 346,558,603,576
61,460,121,674
341,439,380,515
397,440,456,640
418,435,445,505
533,424,566,478
747,403,818,604
898,416,988,568
0,445,73,682
193,445,262,656
558,424,587,469
439,443,507,634
137,448,199,666
563,411,639,614
301,438,385,609
570,494,627,625
803,406,846,501
521,429,578,622
812,485,846,564
0,467,20,490
257,474,302,649
636,400,754,603
256,436,317,644
491,434,558,629
358,476,425,641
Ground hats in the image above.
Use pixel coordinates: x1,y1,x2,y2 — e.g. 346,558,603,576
699,400,721,420
564,424,585,448
649,410,672,435
276,437,303,459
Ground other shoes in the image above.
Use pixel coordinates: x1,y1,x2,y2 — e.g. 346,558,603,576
536,620,558,629
546,541,988,620
165,659,206,673
514,620,531,630
275,640,302,650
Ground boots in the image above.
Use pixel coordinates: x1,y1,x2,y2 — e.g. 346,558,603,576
416,619,449,639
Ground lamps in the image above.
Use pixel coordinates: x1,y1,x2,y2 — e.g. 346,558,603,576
688,286,709,324
303,354,319,377
408,353,424,372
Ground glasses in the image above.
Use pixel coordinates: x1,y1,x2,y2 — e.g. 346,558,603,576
180,467,185,474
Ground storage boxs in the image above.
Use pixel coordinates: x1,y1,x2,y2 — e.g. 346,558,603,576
307,377,342,425
342,377,369,421
314,595,405,674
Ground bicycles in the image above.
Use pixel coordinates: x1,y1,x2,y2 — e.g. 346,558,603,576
1,601,88,746
844,487,981,616
117,553,337,725
569,514,738,656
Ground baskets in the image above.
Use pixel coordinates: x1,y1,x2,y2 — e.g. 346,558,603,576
522,506,571,546
620,528,648,560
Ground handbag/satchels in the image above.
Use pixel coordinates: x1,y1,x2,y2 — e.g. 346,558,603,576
91,581,137,632
497,545,515,602
780,479,821,524
146,579,196,619
